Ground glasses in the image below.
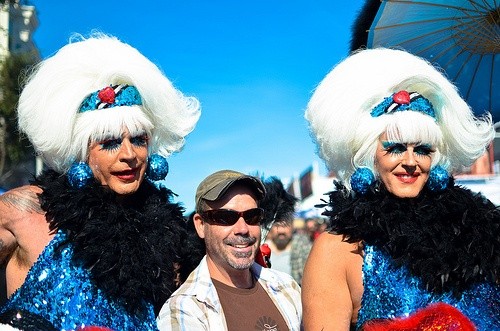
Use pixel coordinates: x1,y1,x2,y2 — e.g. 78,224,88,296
198,207,265,226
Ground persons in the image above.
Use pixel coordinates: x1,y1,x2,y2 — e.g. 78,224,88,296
0,28,201,331
156,170,303,331
262,215,331,286
301,47,500,331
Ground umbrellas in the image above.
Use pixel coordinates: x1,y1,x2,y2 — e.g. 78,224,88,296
348,0,500,126
